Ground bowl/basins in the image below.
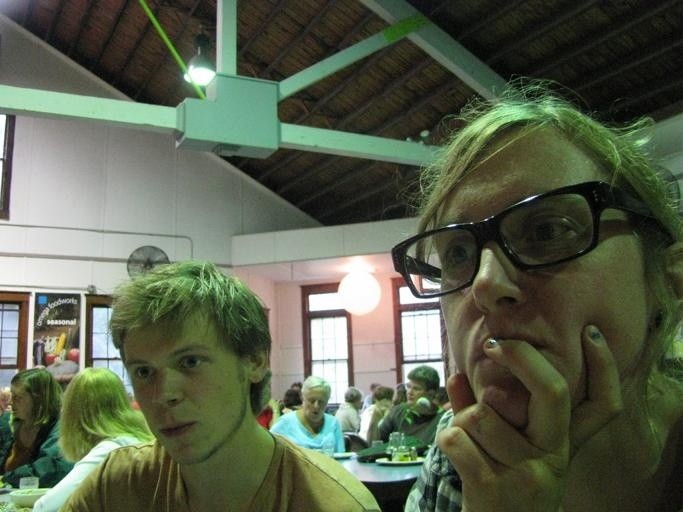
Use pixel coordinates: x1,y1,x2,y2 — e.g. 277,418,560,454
8,487,50,506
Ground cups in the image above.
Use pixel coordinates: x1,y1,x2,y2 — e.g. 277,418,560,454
323,437,333,458
19,475,38,488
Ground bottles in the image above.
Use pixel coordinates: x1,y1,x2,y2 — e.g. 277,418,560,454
386,432,418,461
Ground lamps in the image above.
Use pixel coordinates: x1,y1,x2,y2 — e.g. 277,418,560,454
184,24,216,86
336,272,381,317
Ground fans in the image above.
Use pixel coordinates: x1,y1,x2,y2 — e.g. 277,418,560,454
125,244,170,285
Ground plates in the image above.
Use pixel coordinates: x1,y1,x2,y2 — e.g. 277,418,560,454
376,456,425,465
332,451,354,459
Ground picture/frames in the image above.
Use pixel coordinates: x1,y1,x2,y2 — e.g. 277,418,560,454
22,288,87,382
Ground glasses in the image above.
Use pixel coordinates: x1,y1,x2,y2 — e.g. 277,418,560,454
391,180,658,298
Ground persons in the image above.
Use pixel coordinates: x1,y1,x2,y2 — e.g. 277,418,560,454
268,364,449,453
0,387,10,417
0,367,73,487
32,367,154,511
391,73,683,511
59,258,380,511
